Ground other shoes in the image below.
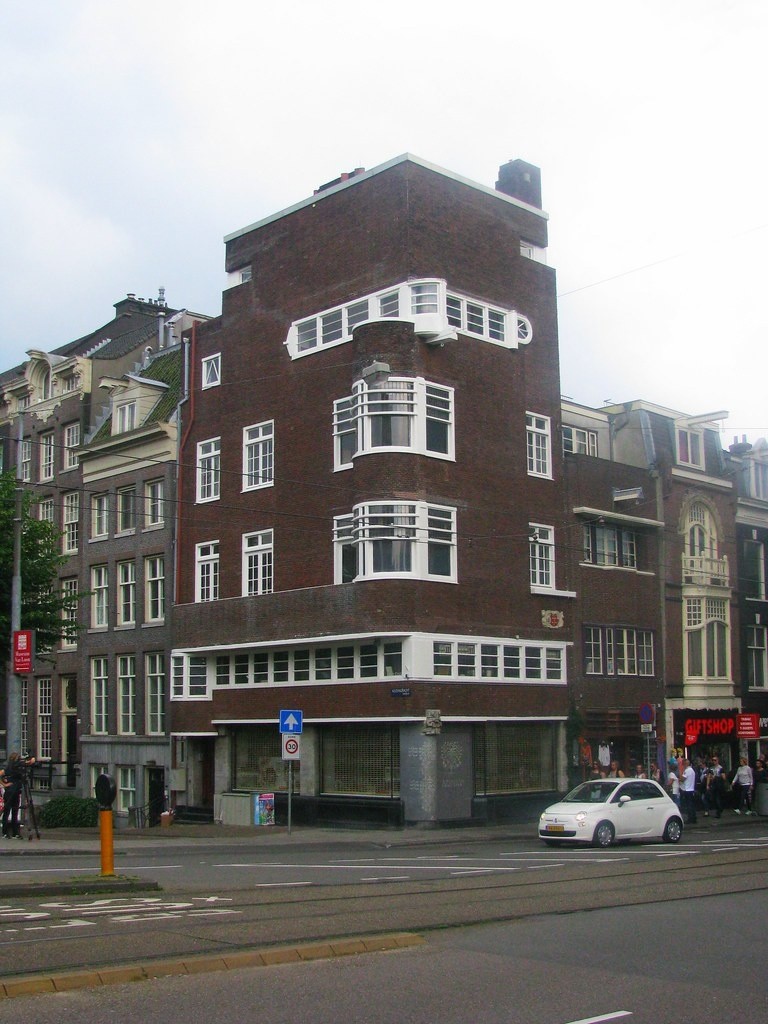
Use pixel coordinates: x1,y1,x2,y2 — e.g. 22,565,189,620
684,809,753,824
2,834,23,840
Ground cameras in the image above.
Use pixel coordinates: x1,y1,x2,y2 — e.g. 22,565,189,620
17,748,35,766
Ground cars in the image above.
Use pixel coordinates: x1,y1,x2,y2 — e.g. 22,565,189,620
536,777,684,848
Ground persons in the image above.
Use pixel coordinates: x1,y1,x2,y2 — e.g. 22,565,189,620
708,756,726,818
699,759,713,816
666,763,681,812
607,760,626,778
649,762,665,789
589,758,606,781
0,752,23,840
752,753,768,817
731,756,754,815
679,759,698,825
634,764,647,779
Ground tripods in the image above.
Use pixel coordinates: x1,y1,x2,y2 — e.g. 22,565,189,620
0,775,41,842
